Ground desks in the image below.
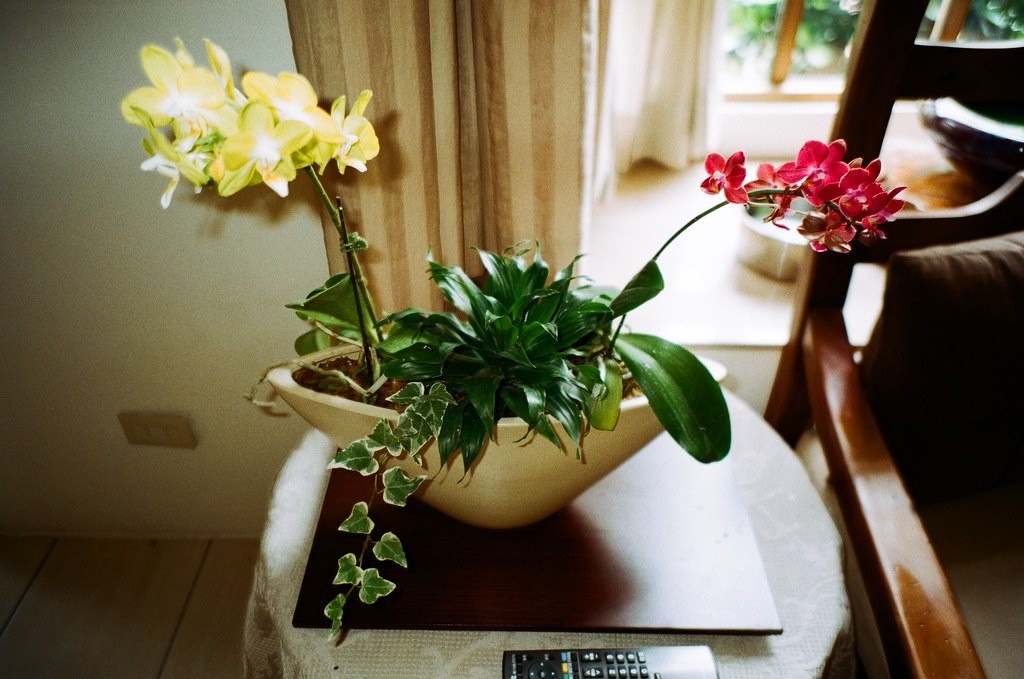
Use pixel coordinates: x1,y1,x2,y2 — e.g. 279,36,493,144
238,379,863,676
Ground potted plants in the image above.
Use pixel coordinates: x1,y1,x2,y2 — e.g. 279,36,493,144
270,315,733,548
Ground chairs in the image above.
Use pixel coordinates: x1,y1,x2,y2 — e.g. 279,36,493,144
759,10,1024,677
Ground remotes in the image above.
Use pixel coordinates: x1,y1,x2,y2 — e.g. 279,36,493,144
502,643,720,679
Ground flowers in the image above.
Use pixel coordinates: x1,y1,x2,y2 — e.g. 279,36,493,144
109,39,919,446
